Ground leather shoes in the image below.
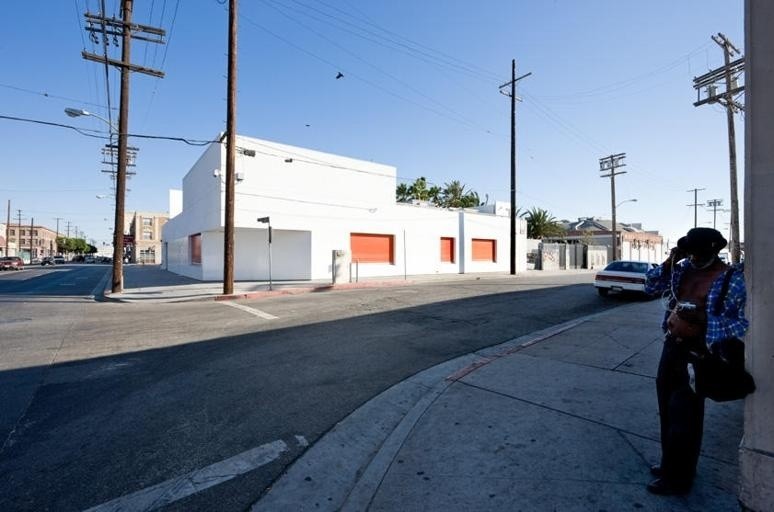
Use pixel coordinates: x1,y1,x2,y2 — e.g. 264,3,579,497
647,464,690,496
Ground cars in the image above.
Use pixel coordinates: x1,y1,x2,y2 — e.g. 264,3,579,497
41,256,53,265
55,254,65,264
1,257,24,271
593,260,659,296
72,255,110,263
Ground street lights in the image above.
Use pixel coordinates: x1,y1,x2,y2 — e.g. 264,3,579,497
65,107,126,294
613,200,636,263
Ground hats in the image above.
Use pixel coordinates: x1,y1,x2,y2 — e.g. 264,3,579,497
677,228,727,252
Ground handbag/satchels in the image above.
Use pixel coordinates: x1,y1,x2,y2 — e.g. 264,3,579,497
700,339,756,403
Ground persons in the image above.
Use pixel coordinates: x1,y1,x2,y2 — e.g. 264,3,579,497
644,226,747,495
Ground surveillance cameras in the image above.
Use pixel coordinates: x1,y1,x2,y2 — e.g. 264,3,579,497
213,169,225,177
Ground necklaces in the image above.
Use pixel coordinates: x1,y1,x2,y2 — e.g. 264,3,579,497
690,253,717,271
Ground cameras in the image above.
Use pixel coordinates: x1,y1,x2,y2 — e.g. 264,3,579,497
671,236,705,259
